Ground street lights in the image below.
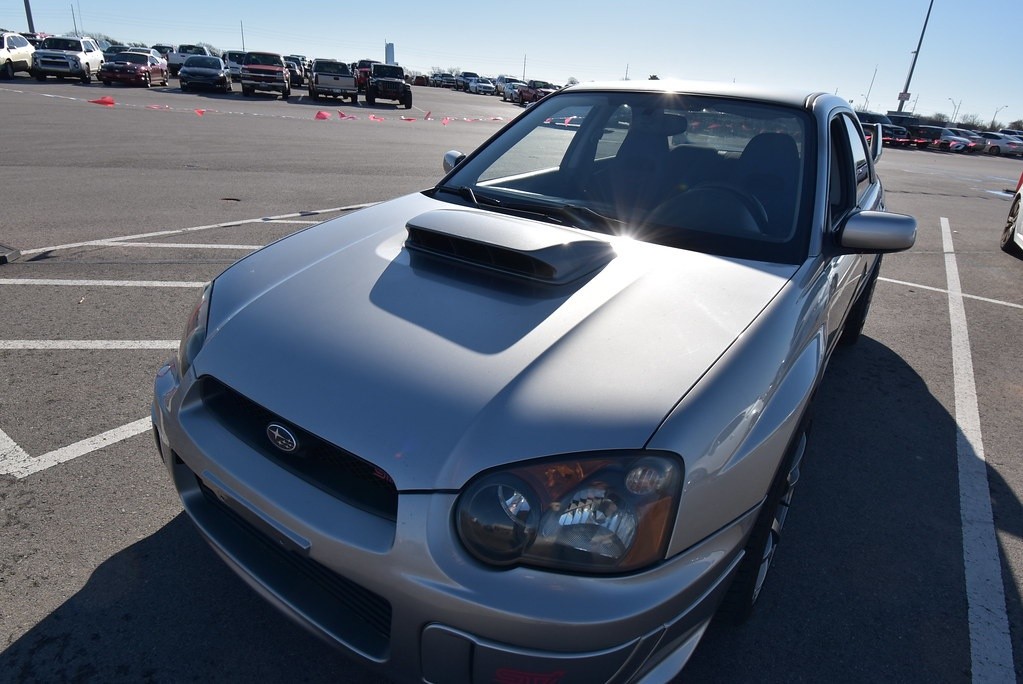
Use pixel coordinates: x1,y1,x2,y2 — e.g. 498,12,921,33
990,105,1009,129
861,93,870,110
948,98,957,123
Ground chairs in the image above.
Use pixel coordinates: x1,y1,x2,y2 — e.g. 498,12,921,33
606,124,801,239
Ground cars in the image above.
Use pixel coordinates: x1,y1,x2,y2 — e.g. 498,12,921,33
18,33,172,61
178,56,233,94
665,110,1023,156
999,176,1023,257
95,53,169,89
149,79,917,684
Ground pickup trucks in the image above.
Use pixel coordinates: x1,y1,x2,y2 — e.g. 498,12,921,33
167,44,212,77
518,80,556,105
306,58,359,104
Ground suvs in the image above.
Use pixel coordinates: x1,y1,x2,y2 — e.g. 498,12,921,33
241,51,292,99
209,50,383,92
32,36,105,85
365,63,412,109
0,32,35,80
411,72,630,125
